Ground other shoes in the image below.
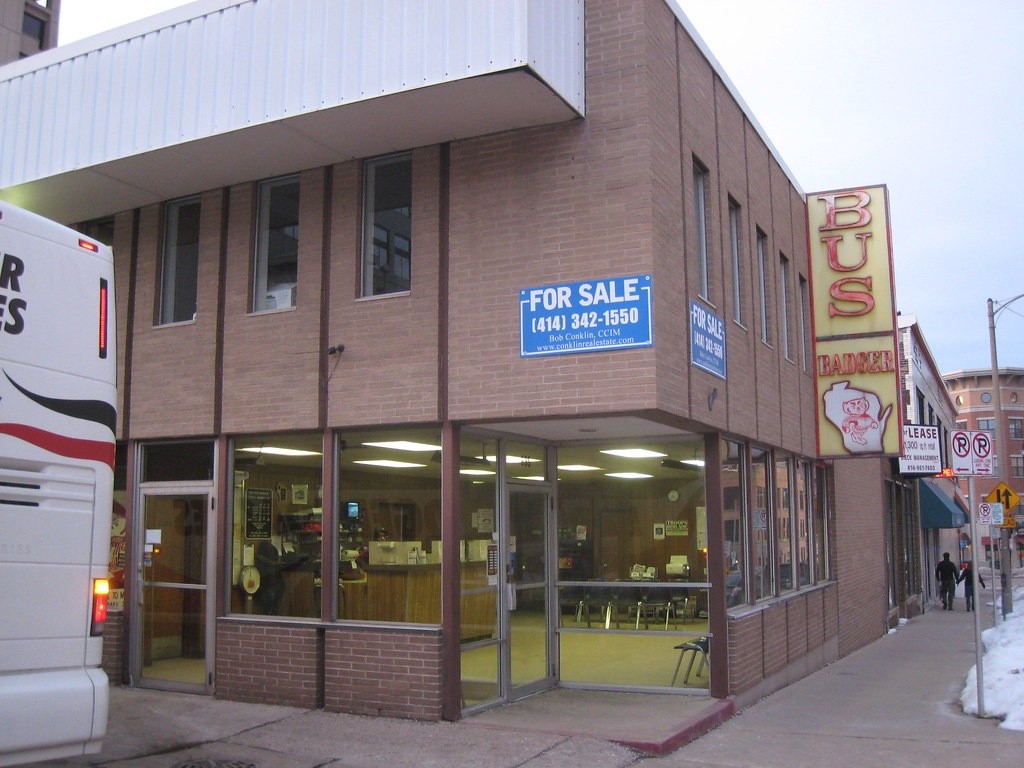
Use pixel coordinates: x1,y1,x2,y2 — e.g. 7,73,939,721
943,605,946,609
948,607,953,610
968,608,971,612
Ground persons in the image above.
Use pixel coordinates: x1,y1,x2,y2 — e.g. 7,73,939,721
936,552,959,610
957,562,985,611
355,527,390,570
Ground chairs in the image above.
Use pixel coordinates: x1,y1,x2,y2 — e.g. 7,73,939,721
559,577,709,688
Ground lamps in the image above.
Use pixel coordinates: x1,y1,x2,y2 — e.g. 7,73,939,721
660,445,702,471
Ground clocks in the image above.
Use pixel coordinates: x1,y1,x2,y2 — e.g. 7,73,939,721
666,488,681,502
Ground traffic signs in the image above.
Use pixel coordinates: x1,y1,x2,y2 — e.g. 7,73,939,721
979,503,1004,526
949,429,994,478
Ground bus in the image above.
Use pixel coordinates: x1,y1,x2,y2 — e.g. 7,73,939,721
0,195,120,768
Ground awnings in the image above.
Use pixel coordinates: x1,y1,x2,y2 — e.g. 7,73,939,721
920,478,965,528
954,490,978,523
